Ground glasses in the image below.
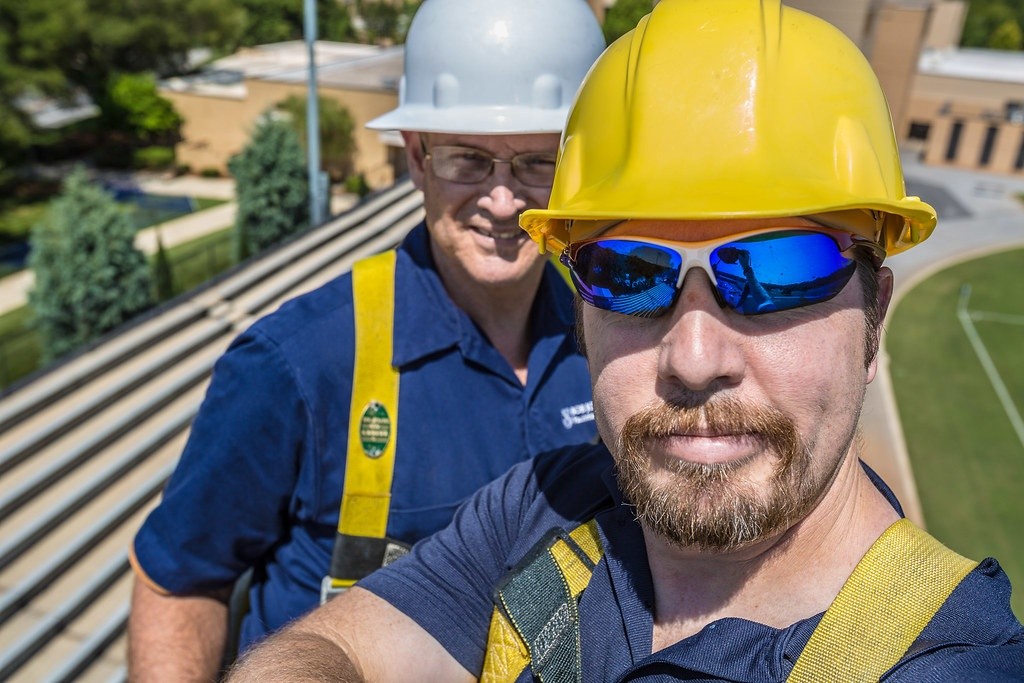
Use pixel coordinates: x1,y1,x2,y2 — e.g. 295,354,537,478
416,137,563,191
555,221,873,317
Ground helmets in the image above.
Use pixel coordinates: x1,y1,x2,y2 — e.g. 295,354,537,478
518,0,939,259
364,0,604,135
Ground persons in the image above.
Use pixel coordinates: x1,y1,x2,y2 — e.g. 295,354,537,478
225,0,1023,683
127,2,598,683
